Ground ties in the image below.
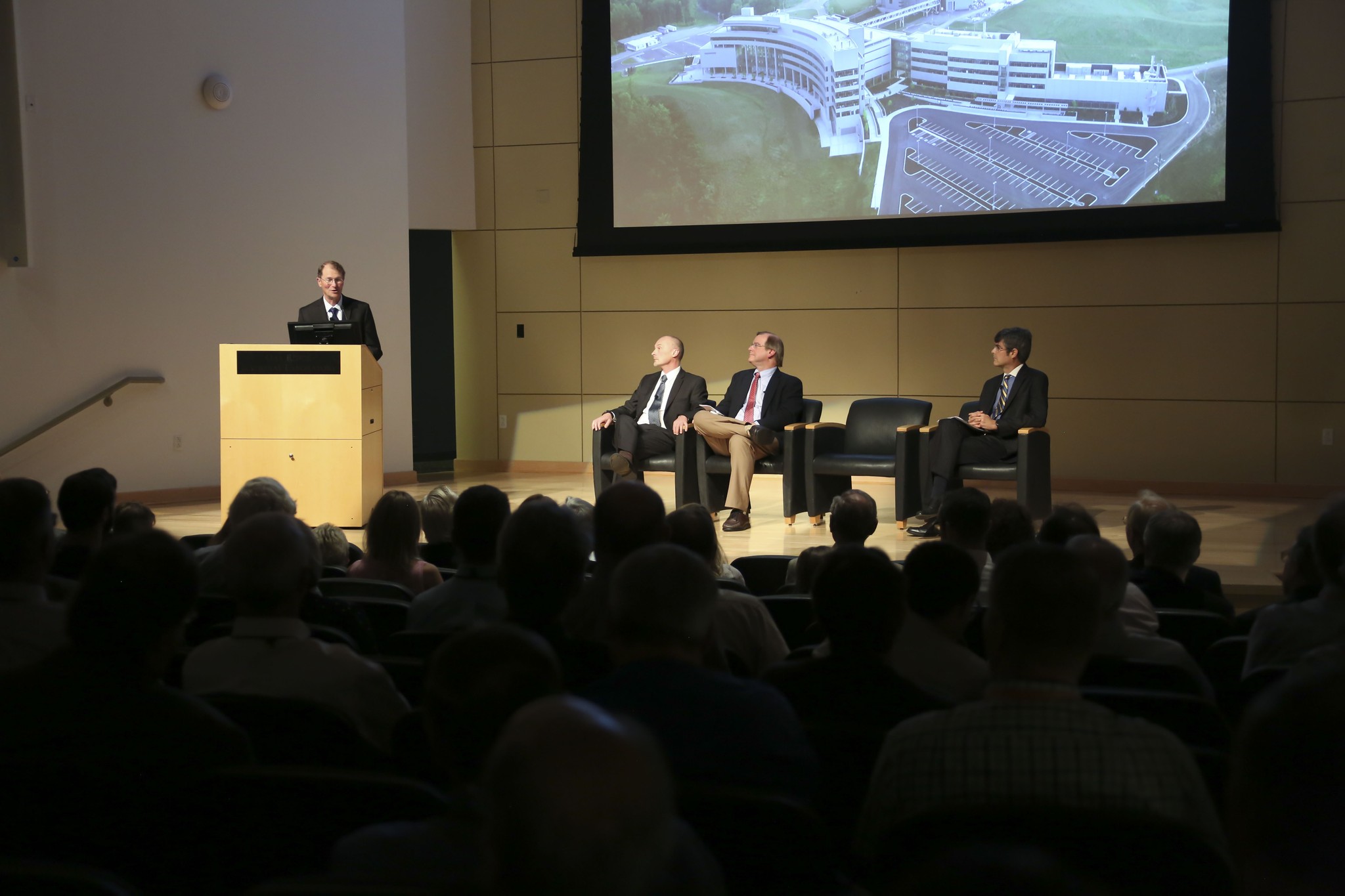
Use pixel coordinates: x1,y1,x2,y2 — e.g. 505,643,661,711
329,307,340,322
744,372,761,425
648,375,667,427
990,374,1012,420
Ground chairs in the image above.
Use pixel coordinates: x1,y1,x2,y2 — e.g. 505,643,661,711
593,400,717,511
696,398,823,526
918,400,1052,532
2,530,1345,896
805,397,934,530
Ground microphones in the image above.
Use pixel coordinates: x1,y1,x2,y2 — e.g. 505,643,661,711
655,394,658,399
340,305,348,320
1000,385,1002,390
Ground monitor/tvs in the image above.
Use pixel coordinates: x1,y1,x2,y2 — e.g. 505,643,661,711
287,320,364,345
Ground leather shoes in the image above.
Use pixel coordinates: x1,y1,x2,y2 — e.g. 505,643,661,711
746,425,775,445
907,523,941,537
722,509,751,531
915,498,941,520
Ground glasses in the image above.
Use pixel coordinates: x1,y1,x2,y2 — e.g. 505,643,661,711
994,345,1006,353
1281,551,1289,562
751,344,778,353
1123,516,1128,525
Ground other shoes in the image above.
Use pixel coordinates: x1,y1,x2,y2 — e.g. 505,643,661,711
610,453,638,481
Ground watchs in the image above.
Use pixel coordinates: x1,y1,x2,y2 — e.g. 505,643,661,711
996,420,999,426
602,410,614,419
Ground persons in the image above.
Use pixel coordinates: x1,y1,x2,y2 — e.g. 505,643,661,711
592,335,708,481
0,469,1345,896
906,326,1050,536
692,333,803,531
298,261,382,361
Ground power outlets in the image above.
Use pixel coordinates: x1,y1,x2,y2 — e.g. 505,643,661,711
499,415,507,429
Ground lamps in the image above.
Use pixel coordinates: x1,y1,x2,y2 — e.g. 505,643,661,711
203,75,233,110
